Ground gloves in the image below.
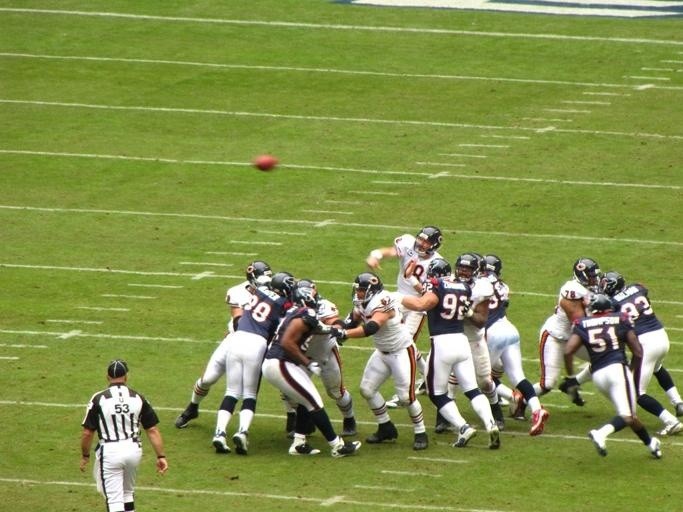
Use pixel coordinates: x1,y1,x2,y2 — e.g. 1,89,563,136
461,299,472,314
306,361,323,377
330,327,346,339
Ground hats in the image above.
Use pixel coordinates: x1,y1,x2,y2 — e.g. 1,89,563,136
107,358,129,379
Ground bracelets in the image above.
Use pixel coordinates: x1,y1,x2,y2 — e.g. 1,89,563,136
157,455,165,459
83,454,89,457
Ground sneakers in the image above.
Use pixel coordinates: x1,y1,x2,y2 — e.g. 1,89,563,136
529,411,549,436
675,401,683,417
175,409,200,428
559,376,584,406
588,430,609,457
655,420,683,437
232,427,249,455
386,395,410,409
212,429,231,453
286,416,506,457
415,381,426,395
511,393,527,420
650,437,662,459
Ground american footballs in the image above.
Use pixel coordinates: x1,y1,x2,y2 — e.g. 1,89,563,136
252,155,277,170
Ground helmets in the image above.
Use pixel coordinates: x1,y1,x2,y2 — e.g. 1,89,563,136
408,227,444,257
422,252,503,282
572,257,626,316
351,272,384,307
245,260,319,309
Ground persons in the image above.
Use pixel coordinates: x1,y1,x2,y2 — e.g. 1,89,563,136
563,292,662,458
80,358,168,512
598,270,683,436
509,257,600,420
175,226,551,459
